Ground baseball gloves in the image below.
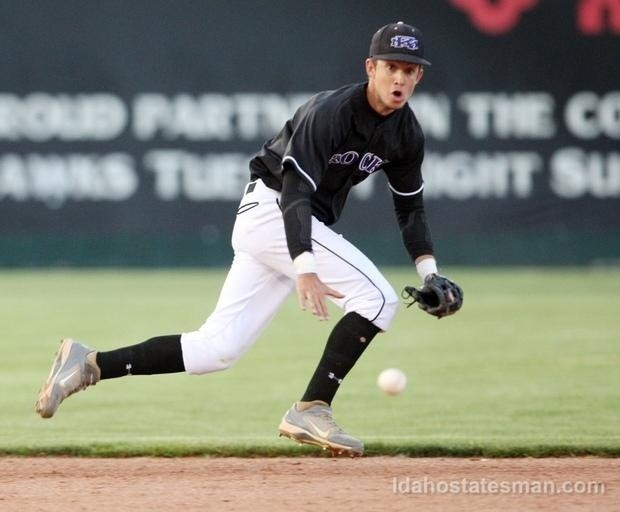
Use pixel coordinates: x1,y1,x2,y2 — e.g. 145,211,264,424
400,275,463,319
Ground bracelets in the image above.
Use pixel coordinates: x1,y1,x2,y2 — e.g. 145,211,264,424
294,251,318,275
415,258,438,279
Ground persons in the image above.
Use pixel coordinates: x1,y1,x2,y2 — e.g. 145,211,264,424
38,21,463,459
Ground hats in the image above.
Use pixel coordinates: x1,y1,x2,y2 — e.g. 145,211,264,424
369,21,432,65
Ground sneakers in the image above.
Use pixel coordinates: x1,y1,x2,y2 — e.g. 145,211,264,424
34,338,96,418
277,403,364,458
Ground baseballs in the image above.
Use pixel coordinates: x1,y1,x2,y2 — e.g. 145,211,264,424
377,369,407,393
